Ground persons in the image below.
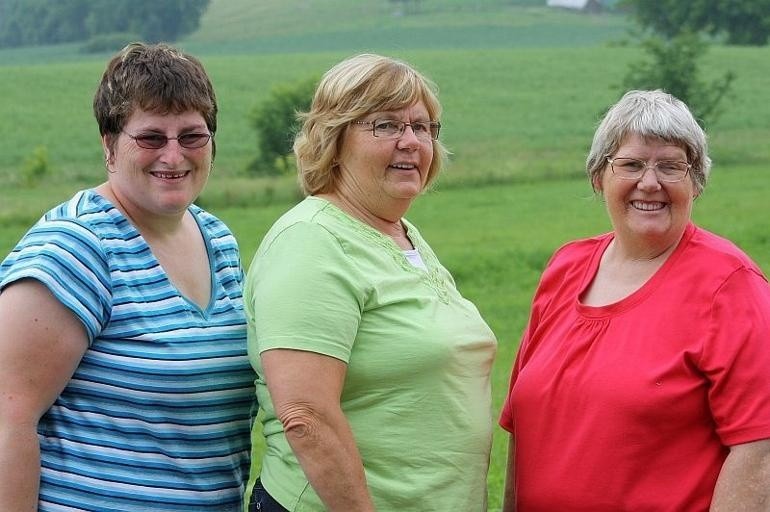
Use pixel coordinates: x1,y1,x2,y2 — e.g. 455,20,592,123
499,88,770,512
240,52,500,512
0,41,258,512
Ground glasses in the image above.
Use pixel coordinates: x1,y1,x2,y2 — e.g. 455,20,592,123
604,153,695,184
112,118,214,151
349,114,442,143
